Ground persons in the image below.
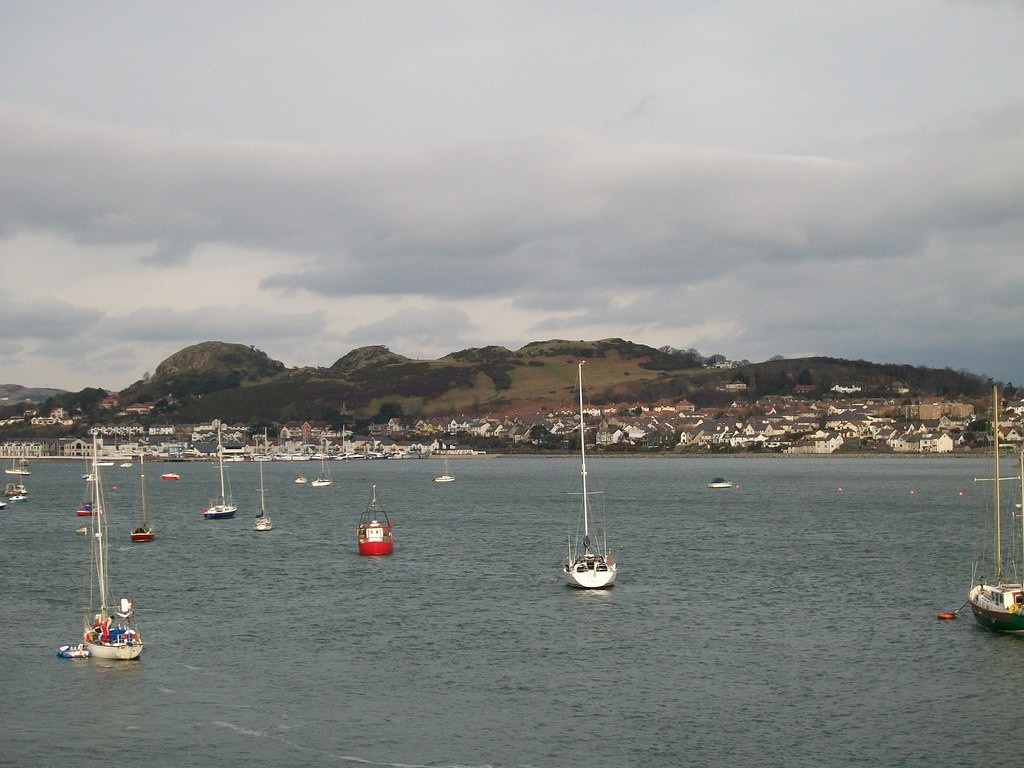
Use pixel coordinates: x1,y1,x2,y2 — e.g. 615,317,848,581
103,615,114,642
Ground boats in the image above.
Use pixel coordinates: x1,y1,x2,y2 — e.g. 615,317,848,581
708,476,734,489
162,472,181,481
293,476,308,484
355,483,396,555
56,645,93,659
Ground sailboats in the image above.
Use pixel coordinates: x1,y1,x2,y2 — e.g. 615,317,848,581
254,458,272,533
966,384,1024,638
203,427,238,521
131,450,155,543
310,436,334,487
76,427,134,518
434,452,455,482
82,429,144,660
1,445,32,509
562,354,617,588
